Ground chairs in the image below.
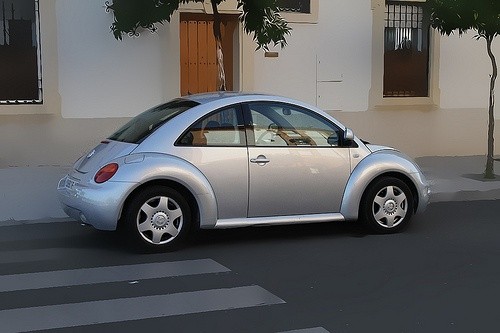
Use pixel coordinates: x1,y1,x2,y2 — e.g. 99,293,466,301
202,119,238,146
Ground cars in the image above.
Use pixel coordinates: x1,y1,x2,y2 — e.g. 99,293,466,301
56,90,431,254
